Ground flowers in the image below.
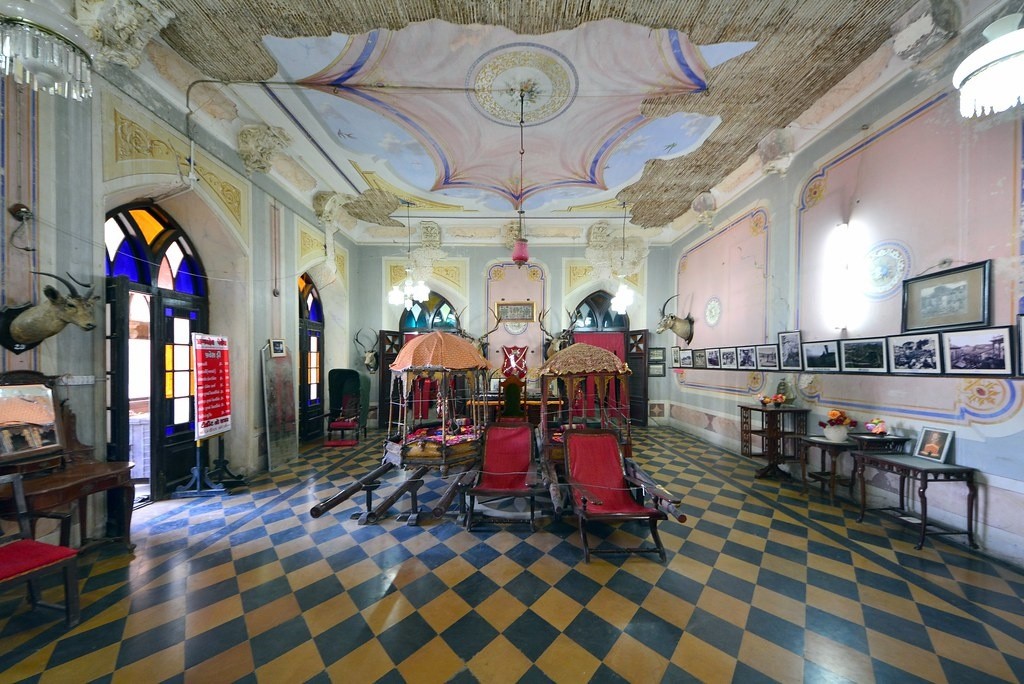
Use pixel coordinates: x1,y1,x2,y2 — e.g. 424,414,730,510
758,392,772,403
816,408,858,430
772,394,786,402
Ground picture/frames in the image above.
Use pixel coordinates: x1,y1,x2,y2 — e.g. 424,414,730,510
911,425,956,464
270,337,287,358
900,258,994,334
737,345,756,372
495,300,536,324
720,346,737,371
693,349,706,369
680,348,693,368
706,347,720,369
756,343,779,372
647,346,667,362
942,324,1016,378
648,362,667,377
802,339,839,374
887,332,942,375
1017,312,1024,377
840,334,887,375
671,346,681,368
777,330,804,372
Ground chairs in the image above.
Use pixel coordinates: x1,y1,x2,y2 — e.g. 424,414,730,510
494,375,529,422
458,420,539,534
0,469,86,631
561,425,669,566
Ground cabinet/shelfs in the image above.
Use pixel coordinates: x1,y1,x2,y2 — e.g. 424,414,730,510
735,402,812,482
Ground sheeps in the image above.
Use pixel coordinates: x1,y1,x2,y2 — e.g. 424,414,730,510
654,292,695,346
8,268,104,346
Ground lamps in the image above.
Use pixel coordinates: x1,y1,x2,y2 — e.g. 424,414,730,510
387,202,431,312
512,154,530,268
952,11,1024,122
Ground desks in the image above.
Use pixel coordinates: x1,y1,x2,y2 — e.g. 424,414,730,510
800,431,983,554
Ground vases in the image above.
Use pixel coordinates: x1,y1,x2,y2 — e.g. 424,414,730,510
821,425,850,442
774,403,781,409
760,401,767,406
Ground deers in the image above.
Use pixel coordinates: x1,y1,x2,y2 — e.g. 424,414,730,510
354,326,382,369
453,304,502,359
536,305,581,359
